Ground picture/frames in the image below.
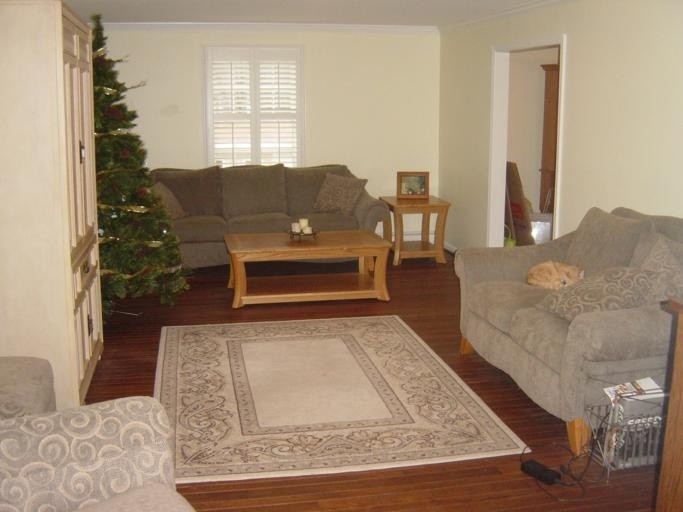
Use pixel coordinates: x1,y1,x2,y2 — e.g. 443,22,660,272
397,171,429,199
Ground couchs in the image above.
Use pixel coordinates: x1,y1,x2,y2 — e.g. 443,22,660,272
126,164,393,273
451,206,681,456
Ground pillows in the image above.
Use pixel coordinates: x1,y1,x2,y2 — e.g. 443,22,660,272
536,267,656,322
149,182,185,220
642,239,682,301
312,171,368,216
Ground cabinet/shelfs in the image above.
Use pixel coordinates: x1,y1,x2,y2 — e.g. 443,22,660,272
2,0,104,408
538,63,559,212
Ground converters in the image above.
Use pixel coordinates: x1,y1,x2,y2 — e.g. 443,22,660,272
521,459,560,483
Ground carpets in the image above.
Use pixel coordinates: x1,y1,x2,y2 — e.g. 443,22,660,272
153,313,531,483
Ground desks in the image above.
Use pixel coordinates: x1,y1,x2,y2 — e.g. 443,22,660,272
379,194,451,265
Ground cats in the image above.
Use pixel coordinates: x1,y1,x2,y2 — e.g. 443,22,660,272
526,259,584,291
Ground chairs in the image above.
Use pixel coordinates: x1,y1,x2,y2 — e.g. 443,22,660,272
0,356,194,512
502,163,550,245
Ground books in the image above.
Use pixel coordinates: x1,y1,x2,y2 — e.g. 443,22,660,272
601,375,669,428
